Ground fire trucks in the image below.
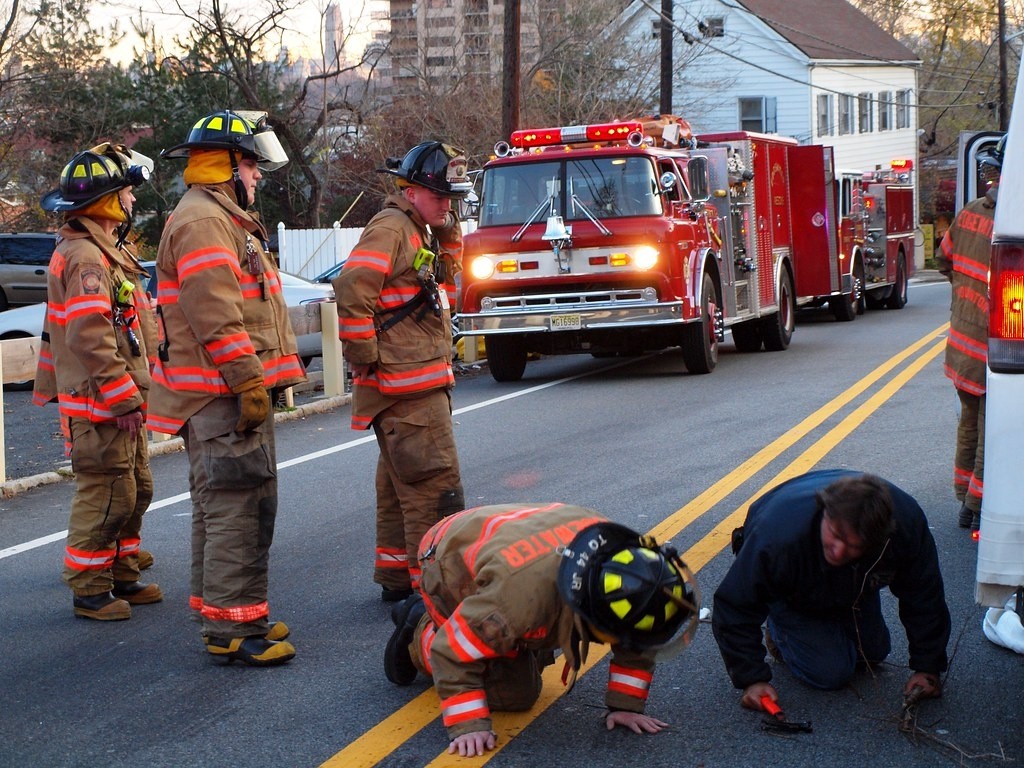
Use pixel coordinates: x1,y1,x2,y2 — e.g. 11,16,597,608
795,161,916,321
453,114,841,384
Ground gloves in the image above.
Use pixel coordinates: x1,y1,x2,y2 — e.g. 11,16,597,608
235,386,270,433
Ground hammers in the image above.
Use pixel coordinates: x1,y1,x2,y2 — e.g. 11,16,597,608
759,694,812,735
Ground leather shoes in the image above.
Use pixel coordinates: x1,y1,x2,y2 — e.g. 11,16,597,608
384,594,426,685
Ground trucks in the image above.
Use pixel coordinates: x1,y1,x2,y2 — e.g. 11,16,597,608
956,53,1024,607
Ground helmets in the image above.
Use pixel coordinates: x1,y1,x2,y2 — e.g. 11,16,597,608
40,151,130,212
556,522,688,644
162,110,261,161
973,132,1007,166
377,141,471,198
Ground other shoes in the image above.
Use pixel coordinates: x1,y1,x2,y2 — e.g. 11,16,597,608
203,622,290,645
957,505,972,526
382,587,414,602
110,580,162,603
208,635,295,664
72,589,133,619
971,513,981,529
138,550,154,569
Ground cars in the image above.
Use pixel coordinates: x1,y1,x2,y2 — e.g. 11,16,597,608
0,231,59,313
312,260,344,284
0,260,336,393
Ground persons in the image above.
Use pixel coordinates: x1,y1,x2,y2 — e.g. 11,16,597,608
331,141,473,604
144,110,310,666
934,131,1010,536
711,469,952,713
31,141,163,621
383,501,702,758
